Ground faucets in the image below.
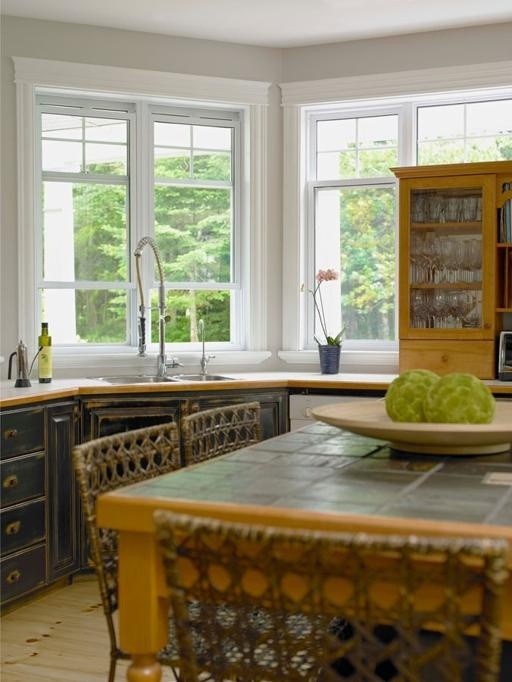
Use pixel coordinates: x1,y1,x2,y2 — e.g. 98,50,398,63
197,319,216,376
133,236,168,376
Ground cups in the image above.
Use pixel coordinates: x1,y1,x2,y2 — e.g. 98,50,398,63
410,195,484,224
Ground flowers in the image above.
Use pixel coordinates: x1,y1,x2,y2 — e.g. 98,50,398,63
302,269,347,346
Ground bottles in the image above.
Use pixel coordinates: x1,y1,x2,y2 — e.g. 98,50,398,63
37,322,52,384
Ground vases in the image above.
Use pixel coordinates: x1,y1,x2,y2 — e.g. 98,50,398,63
319,345,341,374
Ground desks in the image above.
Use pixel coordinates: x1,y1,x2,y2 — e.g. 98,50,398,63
93,422,510,680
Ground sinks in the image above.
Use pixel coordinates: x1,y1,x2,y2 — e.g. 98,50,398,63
97,376,177,384
174,374,227,382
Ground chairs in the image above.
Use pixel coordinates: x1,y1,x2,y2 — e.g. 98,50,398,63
72,420,194,681
180,404,263,469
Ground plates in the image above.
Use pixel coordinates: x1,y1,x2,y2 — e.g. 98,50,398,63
305,400,512,457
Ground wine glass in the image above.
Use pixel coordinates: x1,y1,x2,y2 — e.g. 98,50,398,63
408,229,485,285
410,289,479,330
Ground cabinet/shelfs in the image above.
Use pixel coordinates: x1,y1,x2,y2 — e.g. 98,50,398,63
390,164,496,381
496,242,512,313
82,386,285,574
0,393,79,607
